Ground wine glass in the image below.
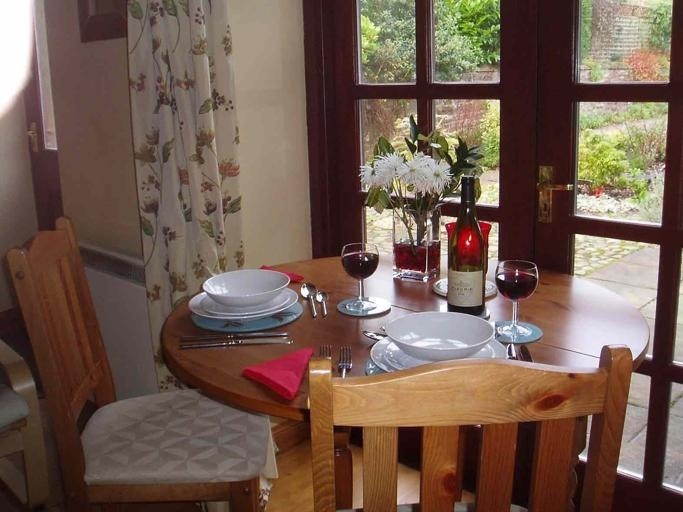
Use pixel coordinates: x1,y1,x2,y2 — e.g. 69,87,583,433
339,243,379,312
493,260,537,338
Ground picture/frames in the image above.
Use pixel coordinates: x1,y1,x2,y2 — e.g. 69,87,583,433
76,1,126,45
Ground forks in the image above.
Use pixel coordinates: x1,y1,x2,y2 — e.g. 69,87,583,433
306,345,331,409
337,343,352,378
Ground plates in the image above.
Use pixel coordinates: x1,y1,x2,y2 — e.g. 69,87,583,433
368,336,508,373
187,287,297,319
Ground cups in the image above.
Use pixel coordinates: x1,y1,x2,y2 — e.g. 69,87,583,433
445,221,491,276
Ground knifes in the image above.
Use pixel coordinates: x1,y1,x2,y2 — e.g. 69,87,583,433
362,330,385,339
179,331,288,341
179,338,293,350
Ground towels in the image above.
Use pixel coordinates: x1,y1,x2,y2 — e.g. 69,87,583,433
250,260,307,289
239,343,318,405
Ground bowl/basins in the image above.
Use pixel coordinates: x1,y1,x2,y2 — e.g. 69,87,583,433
202,269,290,306
385,311,497,361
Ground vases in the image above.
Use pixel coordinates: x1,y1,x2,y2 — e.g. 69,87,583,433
389,200,446,284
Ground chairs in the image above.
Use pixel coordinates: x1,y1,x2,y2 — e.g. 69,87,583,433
300,340,637,510
1,213,283,512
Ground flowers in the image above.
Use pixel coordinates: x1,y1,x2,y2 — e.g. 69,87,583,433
360,116,483,253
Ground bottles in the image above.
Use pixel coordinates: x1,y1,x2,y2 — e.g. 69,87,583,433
445,176,486,315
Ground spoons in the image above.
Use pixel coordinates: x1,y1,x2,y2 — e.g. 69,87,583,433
316,289,327,317
300,282,317,318
